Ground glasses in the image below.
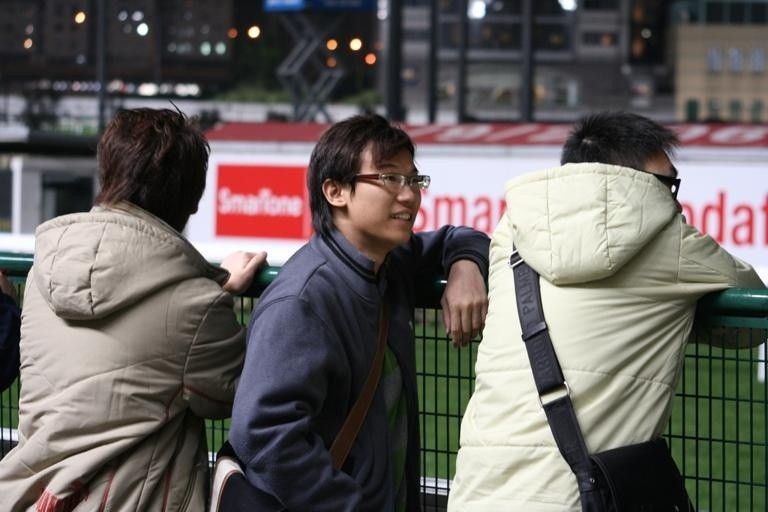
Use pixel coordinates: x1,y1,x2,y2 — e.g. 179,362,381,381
340,171,431,195
652,173,682,202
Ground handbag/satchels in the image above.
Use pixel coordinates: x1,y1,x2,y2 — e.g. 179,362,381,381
206,438,285,512
576,437,697,510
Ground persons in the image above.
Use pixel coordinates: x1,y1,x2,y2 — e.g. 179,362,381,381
0,106,269,511
228,114,489,512
443,105,765,511
0,268,22,389
